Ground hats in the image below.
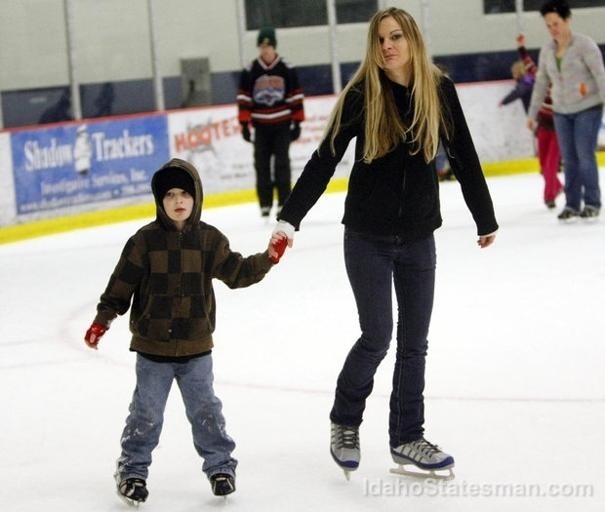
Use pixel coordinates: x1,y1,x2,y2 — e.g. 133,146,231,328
258,26,277,47
158,169,195,207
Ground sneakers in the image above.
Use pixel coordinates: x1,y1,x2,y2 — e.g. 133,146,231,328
390,436,454,468
330,420,360,468
558,209,581,219
209,473,235,496
117,461,148,499
261,207,270,216
579,208,600,218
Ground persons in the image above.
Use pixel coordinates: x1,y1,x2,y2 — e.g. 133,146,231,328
267,6,497,470
238,27,305,217
84,159,288,498
526,1,605,218
39,85,86,124
497,61,564,207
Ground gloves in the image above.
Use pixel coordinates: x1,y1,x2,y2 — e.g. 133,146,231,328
290,127,300,142
242,127,252,143
